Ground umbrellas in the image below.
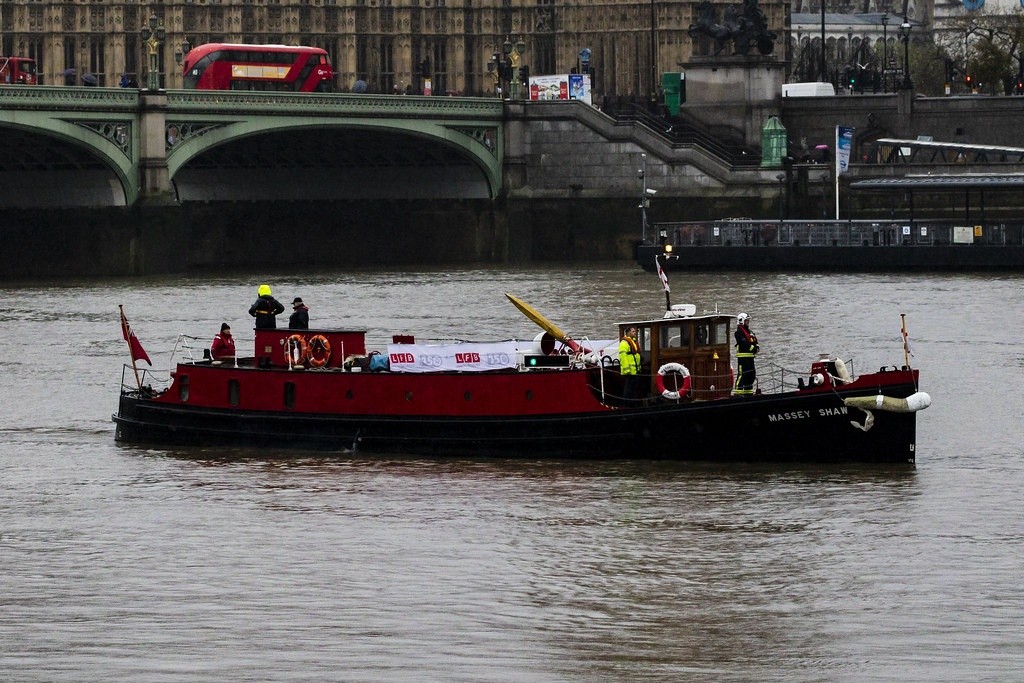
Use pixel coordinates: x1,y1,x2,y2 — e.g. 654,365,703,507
81,74,97,83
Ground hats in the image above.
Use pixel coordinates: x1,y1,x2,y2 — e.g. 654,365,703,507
221,323,230,331
291,298,302,304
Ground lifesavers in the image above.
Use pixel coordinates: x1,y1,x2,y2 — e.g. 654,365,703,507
655,362,692,400
283,334,307,366
306,335,331,368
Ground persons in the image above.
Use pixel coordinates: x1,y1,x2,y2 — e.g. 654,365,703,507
120,74,129,88
734,312,760,398
289,297,309,329
211,323,235,360
249,284,285,328
619,327,641,397
84,82,97,86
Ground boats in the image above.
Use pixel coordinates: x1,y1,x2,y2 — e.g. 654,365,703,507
635,131,1017,272
116,259,929,468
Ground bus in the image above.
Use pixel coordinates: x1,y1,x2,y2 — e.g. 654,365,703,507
182,39,333,101
0,57,37,85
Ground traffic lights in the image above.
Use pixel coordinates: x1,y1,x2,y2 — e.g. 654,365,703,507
945,60,956,84
847,67,856,84
516,64,528,85
965,70,972,86
421,57,431,78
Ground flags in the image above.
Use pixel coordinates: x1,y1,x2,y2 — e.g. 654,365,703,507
656,258,671,294
121,311,152,366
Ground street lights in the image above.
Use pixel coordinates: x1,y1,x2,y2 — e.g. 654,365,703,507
515,36,526,67
880,13,890,89
901,16,916,88
143,16,166,89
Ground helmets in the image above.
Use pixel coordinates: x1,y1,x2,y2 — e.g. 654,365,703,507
258,285,271,297
737,313,750,326
